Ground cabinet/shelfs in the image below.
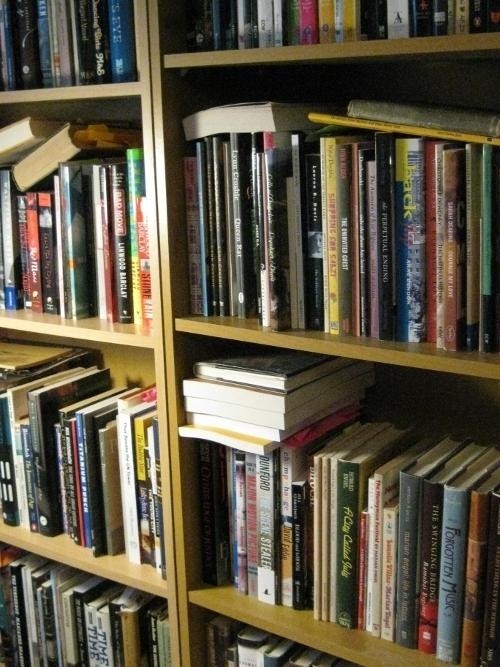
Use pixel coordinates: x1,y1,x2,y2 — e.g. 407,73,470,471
0,0,184,667
149,0,496,666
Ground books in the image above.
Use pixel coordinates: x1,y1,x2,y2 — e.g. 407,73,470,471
0,0,139,93
203,614,358,667
0,335,167,583
196,0,499,53
177,98,500,357
0,115,153,331
0,545,173,667
177,358,500,667
